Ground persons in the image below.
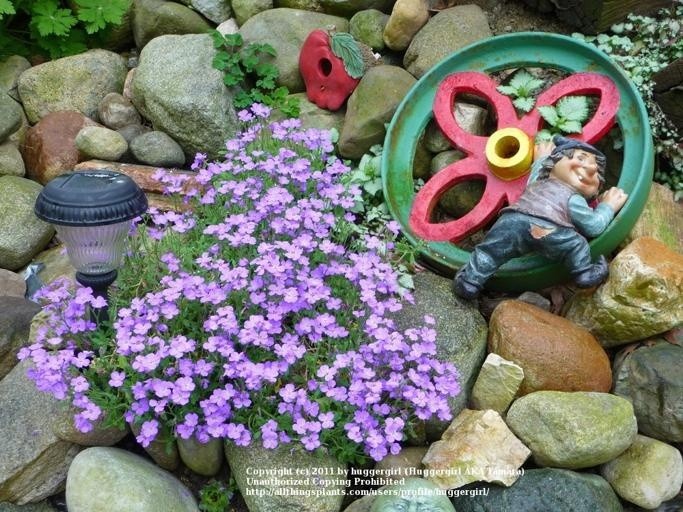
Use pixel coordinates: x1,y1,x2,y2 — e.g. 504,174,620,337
454,140,626,295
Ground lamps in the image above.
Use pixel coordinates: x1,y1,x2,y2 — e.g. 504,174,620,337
33,168,149,325
